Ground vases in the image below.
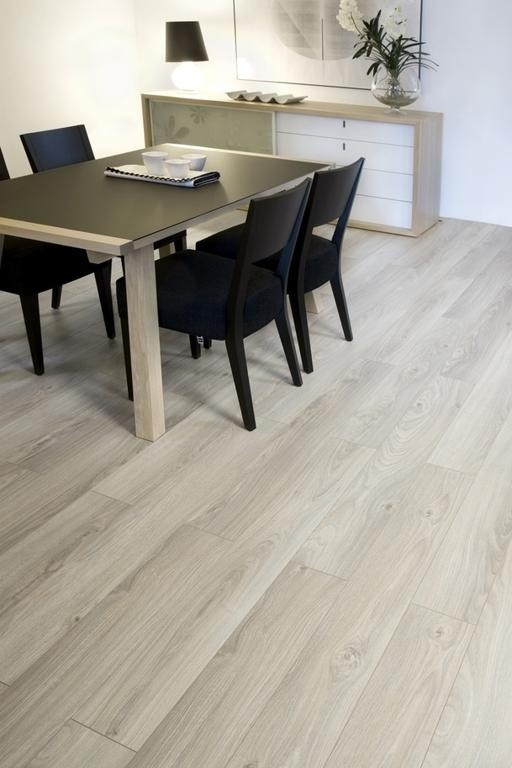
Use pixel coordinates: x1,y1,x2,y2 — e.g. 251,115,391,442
368,65,421,111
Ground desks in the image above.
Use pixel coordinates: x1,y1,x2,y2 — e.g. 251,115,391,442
0,143,346,402
142,91,445,237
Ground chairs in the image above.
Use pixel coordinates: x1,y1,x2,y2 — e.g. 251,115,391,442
112,170,312,430
21,123,192,338
200,151,367,373
0,149,117,379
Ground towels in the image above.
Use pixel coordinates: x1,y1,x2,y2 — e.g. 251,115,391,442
104,162,222,190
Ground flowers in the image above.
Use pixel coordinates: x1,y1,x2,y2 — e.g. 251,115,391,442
332,0,443,75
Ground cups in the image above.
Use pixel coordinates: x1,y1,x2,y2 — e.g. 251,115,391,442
186,152,210,173
164,158,190,181
140,150,172,178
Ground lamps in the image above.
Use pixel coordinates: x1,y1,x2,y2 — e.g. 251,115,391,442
164,19,210,94
